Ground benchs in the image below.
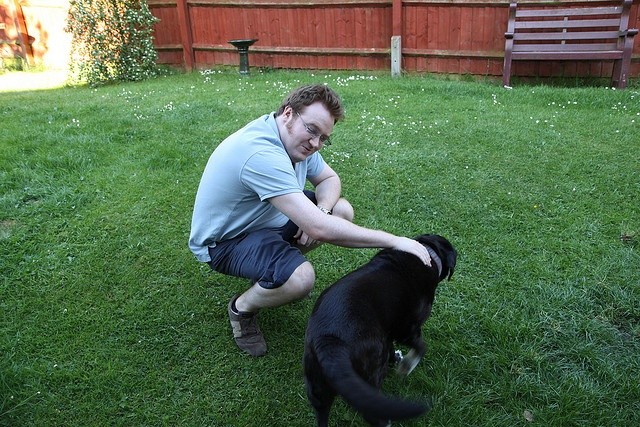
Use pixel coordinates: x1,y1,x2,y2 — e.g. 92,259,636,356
502,0,639,89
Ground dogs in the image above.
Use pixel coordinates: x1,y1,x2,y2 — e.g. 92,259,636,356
302,233,457,426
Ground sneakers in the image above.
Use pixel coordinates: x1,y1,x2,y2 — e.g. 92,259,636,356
227,293,267,356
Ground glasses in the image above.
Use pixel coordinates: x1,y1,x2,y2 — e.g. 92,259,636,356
294,108,331,146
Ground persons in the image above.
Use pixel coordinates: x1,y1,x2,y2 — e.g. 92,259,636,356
188,84,433,354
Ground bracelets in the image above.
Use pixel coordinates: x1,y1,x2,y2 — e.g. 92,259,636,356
318,204,330,215
